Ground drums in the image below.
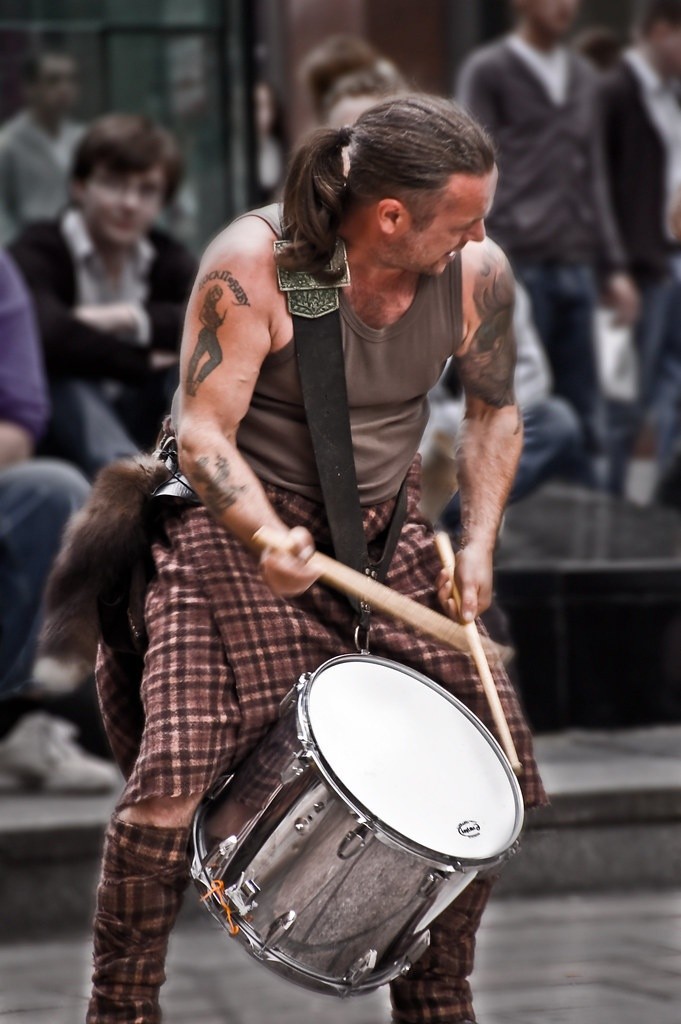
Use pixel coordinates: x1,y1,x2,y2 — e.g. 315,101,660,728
173,645,530,1001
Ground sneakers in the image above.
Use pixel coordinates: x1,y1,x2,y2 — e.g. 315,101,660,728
0,711,119,793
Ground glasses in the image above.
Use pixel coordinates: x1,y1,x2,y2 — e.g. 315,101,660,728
87,171,164,200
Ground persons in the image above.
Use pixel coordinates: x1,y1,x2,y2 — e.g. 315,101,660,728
0,1,681,793
85,93,550,1024
